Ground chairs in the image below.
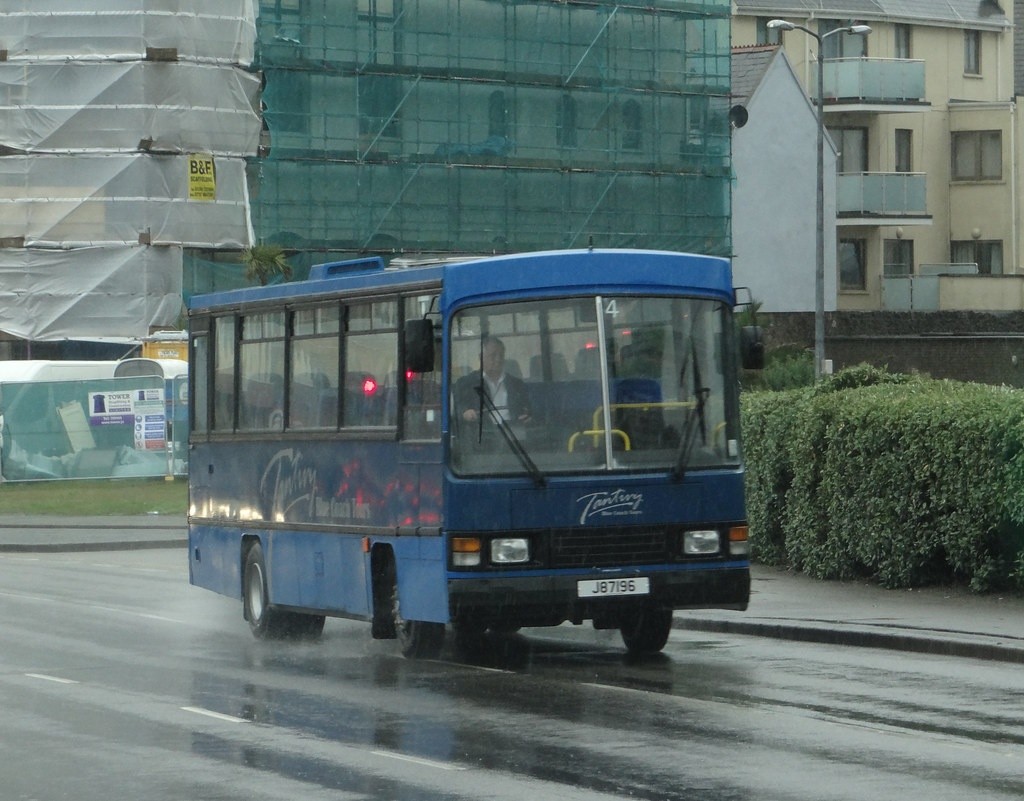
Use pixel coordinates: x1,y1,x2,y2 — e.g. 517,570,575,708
209,369,665,453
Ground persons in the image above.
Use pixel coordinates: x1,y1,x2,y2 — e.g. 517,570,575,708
453,336,537,424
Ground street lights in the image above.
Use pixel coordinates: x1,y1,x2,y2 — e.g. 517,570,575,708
766,18,873,389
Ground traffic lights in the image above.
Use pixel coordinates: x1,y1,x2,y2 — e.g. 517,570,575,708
363,380,376,395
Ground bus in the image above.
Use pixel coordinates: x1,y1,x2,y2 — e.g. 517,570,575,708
187,235,767,658
